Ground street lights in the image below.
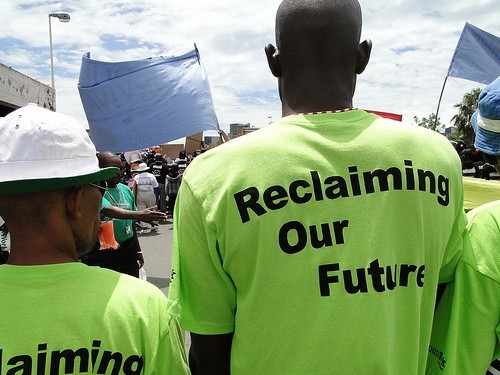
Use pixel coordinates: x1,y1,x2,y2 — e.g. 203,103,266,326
48,12,71,92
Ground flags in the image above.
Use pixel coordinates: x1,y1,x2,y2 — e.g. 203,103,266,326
446,22,500,85
78,48,220,153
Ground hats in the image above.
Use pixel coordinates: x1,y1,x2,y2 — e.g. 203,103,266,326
136,163,150,171
470,76,500,154
169,165,180,171
0,104,120,195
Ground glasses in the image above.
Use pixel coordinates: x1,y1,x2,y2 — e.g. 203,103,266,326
88,181,107,197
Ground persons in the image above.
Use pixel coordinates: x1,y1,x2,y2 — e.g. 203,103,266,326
131,163,159,235
0,103,191,375
81,151,144,278
165,163,183,219
425,76,500,375
166,0,469,375
127,150,199,225
